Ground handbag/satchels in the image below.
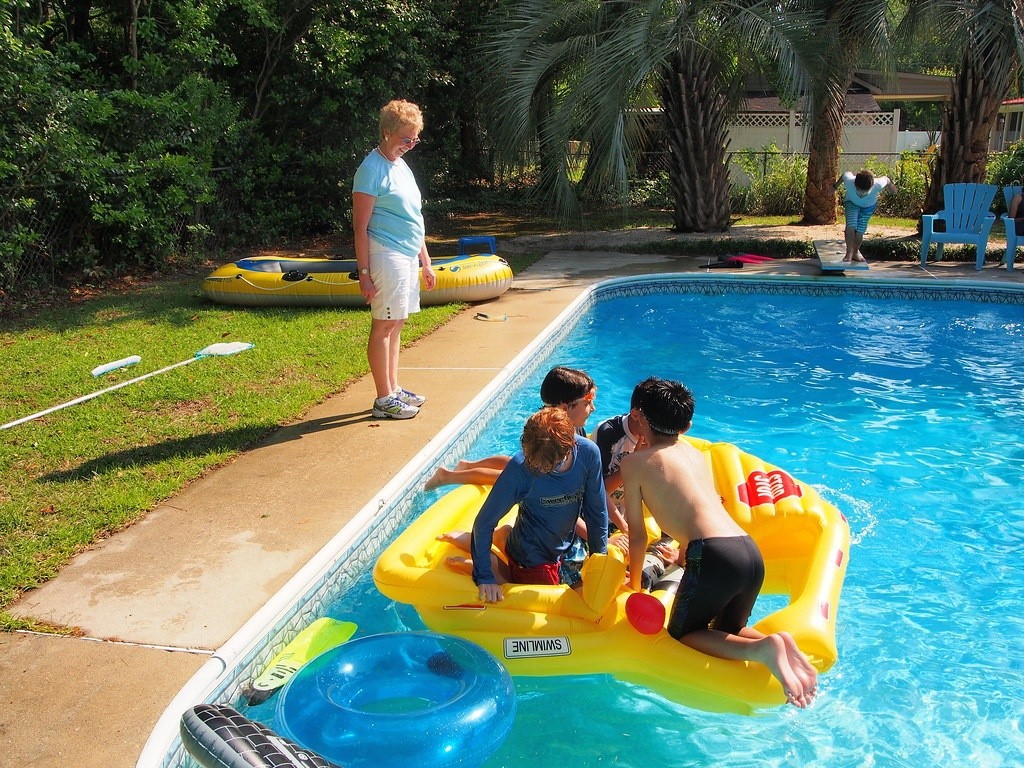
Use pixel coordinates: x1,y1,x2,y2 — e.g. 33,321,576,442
508,558,563,585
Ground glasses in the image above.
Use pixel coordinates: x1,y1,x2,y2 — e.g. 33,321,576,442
526,461,563,473
395,130,422,145
566,386,597,404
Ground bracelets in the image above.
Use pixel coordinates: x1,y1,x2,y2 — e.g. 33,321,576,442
833,183,837,190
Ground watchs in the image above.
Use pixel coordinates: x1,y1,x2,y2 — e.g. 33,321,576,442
358,267,369,276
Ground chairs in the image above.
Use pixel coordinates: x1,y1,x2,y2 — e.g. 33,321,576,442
919,182,998,269
999,185,1024,273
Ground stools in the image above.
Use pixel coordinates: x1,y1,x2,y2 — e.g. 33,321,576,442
456,236,495,252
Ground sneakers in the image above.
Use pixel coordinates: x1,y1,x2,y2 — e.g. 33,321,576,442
392,385,426,407
372,387,420,419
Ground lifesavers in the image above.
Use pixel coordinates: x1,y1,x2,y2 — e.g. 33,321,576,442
272,633,518,767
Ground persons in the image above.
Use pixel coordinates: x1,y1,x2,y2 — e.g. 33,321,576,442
1007,183,1024,236
827,168,897,262
433,407,611,604
621,379,819,708
423,372,678,541
491,366,632,598
351,100,437,421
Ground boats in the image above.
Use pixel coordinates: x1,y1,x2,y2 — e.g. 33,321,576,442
204,255,516,304
